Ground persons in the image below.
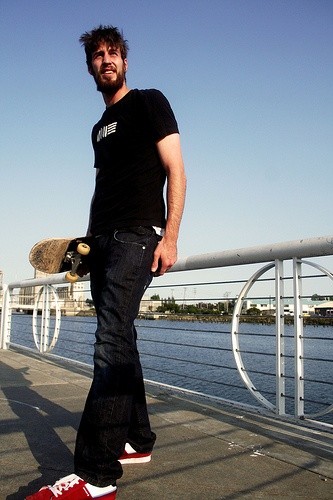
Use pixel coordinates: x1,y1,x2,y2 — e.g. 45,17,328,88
26,24,187,500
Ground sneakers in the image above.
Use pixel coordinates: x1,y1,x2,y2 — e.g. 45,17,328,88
26,473,117,500
118,443,152,465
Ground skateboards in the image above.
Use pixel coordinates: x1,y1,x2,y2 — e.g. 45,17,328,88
28,234,91,283
